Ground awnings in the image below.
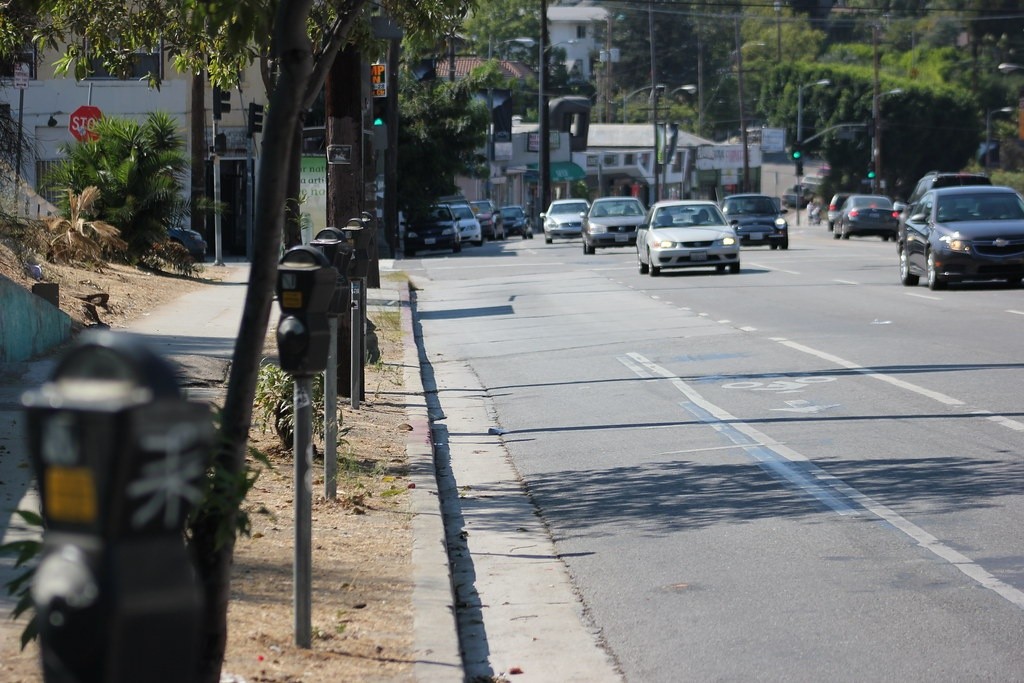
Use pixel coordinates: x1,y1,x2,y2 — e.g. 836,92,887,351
524,162,587,185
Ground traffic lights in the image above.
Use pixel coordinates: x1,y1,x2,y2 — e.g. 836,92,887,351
246,102,263,139
374,98,384,126
212,82,230,120
868,162,875,178
214,133,227,155
790,139,803,176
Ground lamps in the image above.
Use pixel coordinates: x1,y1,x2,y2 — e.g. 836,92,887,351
48,111,63,127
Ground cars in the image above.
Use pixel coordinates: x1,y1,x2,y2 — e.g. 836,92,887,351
540,198,595,244
400,205,462,257
825,193,911,241
717,194,788,250
580,198,648,255
636,200,740,277
159,219,207,266
898,186,1024,291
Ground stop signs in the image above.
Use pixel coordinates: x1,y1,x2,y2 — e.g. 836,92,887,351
69,105,106,143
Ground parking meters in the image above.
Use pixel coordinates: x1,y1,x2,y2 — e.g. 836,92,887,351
310,211,377,500
20,329,208,682
275,246,333,647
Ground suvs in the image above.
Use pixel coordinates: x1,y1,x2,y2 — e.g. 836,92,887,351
428,195,533,247
892,171,999,260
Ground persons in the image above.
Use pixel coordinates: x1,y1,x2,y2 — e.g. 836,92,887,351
807,195,826,226
655,208,674,226
521,211,535,240
697,208,714,225
487,209,506,241
630,179,641,198
726,203,741,216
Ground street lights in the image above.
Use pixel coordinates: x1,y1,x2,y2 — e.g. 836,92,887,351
621,85,666,126
662,84,697,199
869,89,901,193
536,38,581,231
482,35,535,199
796,80,829,226
983,107,1013,178
